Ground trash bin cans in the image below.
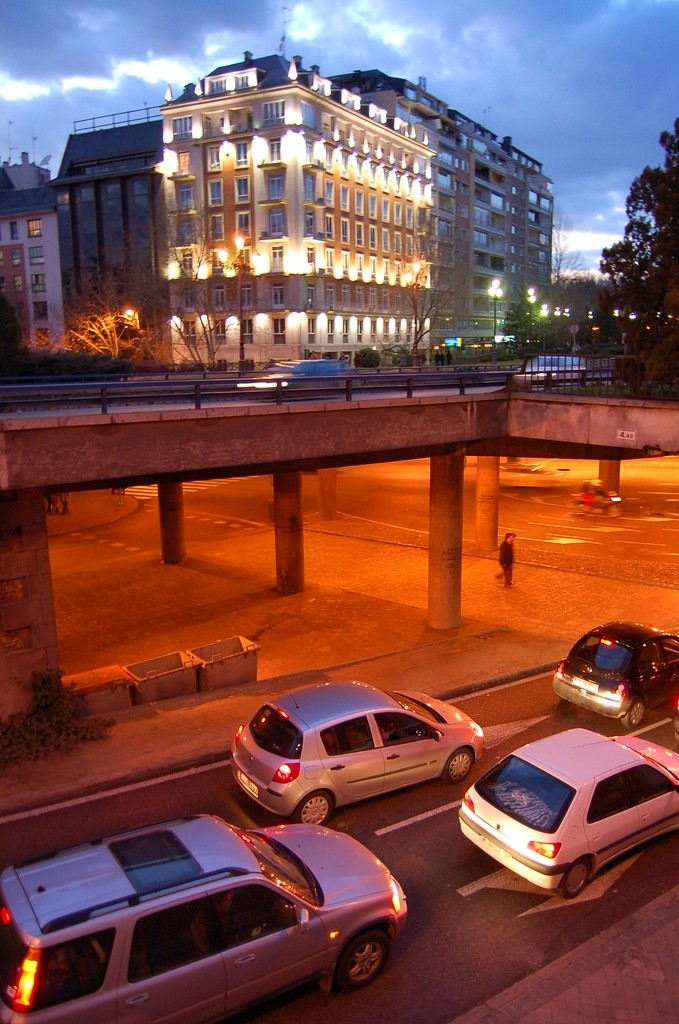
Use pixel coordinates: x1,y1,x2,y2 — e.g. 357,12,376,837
62,664,134,717
122,650,201,707
186,635,262,694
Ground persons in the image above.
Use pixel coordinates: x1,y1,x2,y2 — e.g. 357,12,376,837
496,533,516,589
310,351,318,359
386,354,393,371
112,488,125,495
44,492,70,515
339,353,346,360
434,350,453,371
380,722,398,740
419,351,426,364
579,480,604,509
216,890,262,945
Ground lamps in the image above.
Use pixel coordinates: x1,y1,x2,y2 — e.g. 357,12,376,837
318,267,325,275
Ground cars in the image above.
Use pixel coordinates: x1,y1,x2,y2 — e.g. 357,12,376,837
515,355,587,389
236,360,353,390
552,620,679,728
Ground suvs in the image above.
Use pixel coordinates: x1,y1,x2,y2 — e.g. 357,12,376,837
457,728,679,901
230,678,485,825
0,812,409,1024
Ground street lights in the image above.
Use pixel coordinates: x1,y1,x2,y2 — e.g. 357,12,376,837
214,232,267,365
525,287,594,355
402,262,430,366
487,278,503,363
